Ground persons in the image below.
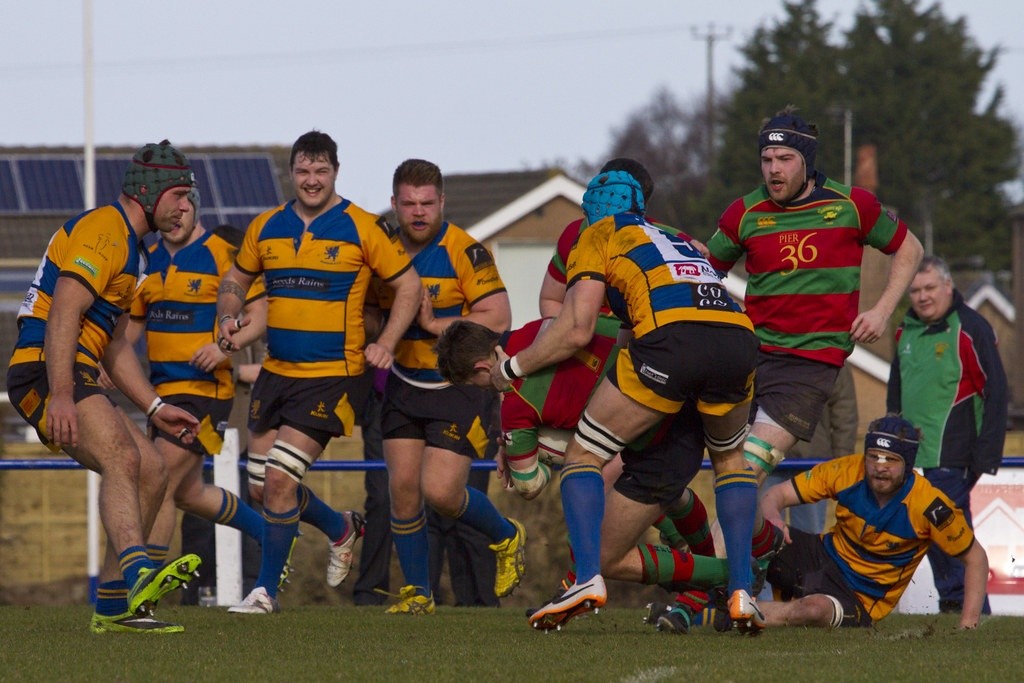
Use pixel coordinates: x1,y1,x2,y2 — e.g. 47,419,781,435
97,109,925,634
885,256,1010,614
644,415,989,633
7,139,201,633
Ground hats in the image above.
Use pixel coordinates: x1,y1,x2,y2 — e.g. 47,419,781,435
759,112,817,185
864,414,920,484
121,137,195,213
189,176,202,226
580,170,645,226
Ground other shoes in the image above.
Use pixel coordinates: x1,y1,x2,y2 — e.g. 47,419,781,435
352,587,401,605
525,584,568,617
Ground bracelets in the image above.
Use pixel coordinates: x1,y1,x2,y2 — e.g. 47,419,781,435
144,395,166,420
501,354,522,381
218,314,232,324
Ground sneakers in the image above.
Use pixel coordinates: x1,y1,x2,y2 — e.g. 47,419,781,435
327,510,367,587
727,590,767,636
126,552,202,617
276,531,303,593
90,608,185,634
643,585,731,634
226,586,279,614
488,518,527,597
384,584,436,614
527,569,607,634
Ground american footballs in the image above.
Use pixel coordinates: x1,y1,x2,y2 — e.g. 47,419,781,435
536,427,572,465
